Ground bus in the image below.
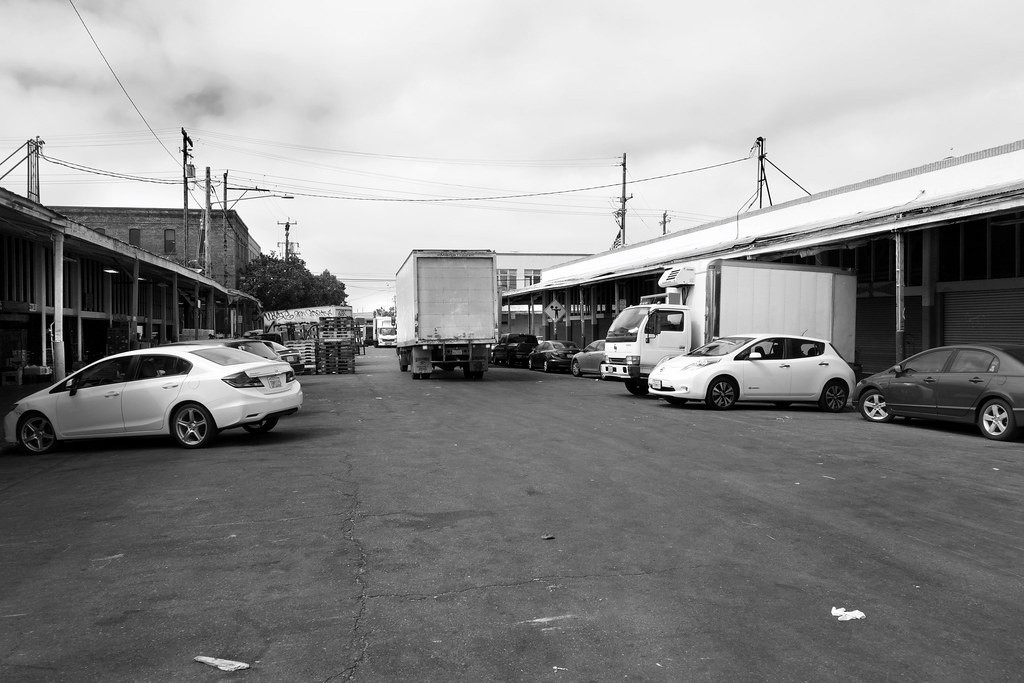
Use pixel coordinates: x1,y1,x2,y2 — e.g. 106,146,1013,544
354,316,367,346
243,329,264,338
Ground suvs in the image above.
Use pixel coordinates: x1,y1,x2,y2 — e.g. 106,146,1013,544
491,333,539,368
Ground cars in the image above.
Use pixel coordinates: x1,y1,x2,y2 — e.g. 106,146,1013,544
159,339,285,363
571,339,606,377
0,343,304,455
254,339,304,373
526,340,581,372
647,332,857,412
852,342,1024,441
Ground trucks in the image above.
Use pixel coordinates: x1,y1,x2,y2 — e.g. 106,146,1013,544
372,316,397,348
599,259,858,398
361,325,373,346
392,248,500,381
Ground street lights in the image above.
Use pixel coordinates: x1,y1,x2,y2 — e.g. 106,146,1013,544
205,194,294,332
